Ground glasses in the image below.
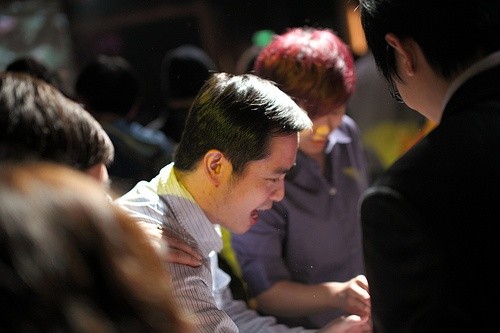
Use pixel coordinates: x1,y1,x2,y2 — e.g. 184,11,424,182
388,73,405,105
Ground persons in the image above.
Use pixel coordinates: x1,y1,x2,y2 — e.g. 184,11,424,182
1,75,112,197
0,158,196,333
229,27,389,333
355,0,500,333
0,45,266,196
114,69,371,333
